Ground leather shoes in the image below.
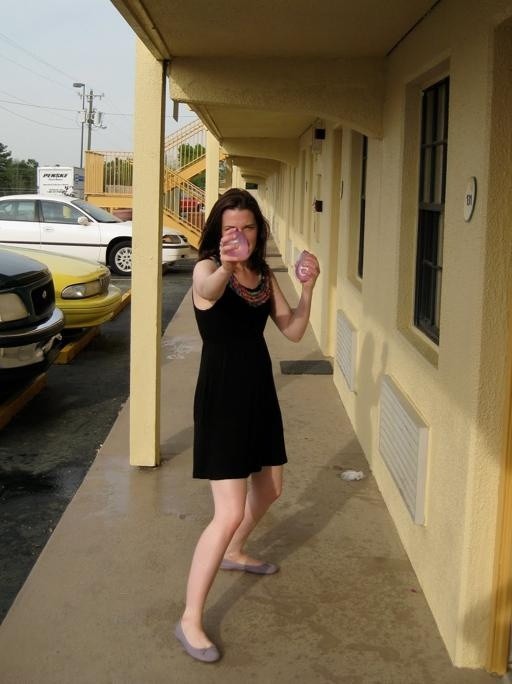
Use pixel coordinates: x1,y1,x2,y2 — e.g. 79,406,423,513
174,618,220,662
221,554,279,575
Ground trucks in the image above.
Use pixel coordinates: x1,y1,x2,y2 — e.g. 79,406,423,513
37,167,84,200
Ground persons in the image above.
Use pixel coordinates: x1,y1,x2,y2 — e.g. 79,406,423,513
170,188,320,664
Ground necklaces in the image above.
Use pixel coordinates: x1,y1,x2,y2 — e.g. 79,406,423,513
214,258,271,307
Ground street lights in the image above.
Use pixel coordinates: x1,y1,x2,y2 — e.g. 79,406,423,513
72,83,87,168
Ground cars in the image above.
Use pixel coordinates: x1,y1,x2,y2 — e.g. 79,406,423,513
0,194,191,401
